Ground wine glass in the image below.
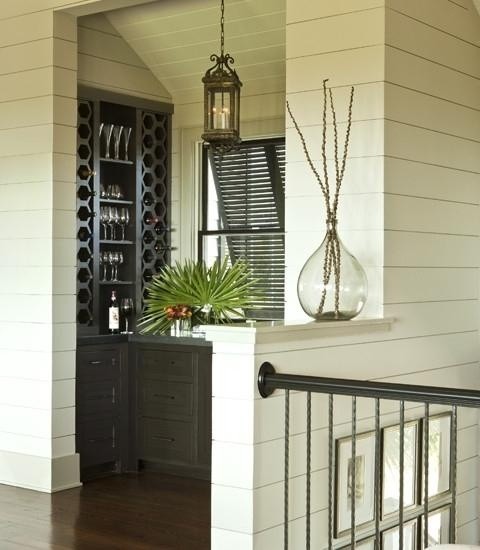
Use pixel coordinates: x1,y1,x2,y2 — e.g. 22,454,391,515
99,252,123,283
121,298,134,335
101,207,129,241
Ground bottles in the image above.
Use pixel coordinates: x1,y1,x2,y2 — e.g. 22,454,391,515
109,291,120,334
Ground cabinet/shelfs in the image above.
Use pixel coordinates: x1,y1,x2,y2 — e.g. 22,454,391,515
77,83,174,334
128,334,211,483
75,335,128,483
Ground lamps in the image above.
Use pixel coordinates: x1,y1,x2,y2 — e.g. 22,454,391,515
200,0,245,157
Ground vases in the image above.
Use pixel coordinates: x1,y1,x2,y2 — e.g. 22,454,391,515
293,215,366,322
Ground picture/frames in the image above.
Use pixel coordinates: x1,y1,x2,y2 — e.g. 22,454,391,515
335,410,455,549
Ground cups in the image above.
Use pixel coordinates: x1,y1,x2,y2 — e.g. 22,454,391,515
99,122,132,160
100,183,120,199
